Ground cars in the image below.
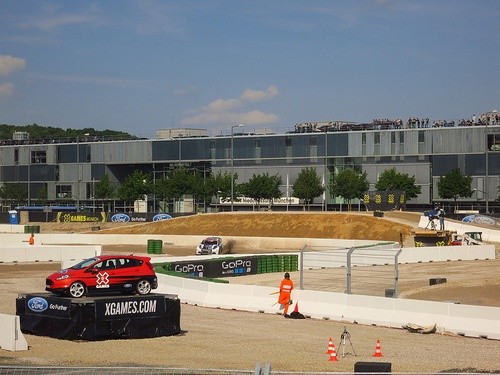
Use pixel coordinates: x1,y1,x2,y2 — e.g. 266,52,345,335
45,254,159,298
195,236,225,256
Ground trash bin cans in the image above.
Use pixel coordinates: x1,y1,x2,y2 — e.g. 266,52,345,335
8,210,19,224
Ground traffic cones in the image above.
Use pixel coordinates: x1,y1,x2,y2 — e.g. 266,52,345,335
325,338,334,354
372,339,384,357
328,343,341,360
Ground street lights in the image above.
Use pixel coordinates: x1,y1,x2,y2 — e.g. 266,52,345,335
231,124,244,212
76,133,90,206
316,128,328,213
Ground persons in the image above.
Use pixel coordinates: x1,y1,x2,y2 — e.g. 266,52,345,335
29,233,34,245
278,272,294,317
443,234,449,246
294,111,500,133
437,207,445,230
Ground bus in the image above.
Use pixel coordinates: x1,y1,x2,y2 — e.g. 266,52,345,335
14,203,109,223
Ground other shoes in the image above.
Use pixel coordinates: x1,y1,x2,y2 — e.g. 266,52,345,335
289,300,292,304
